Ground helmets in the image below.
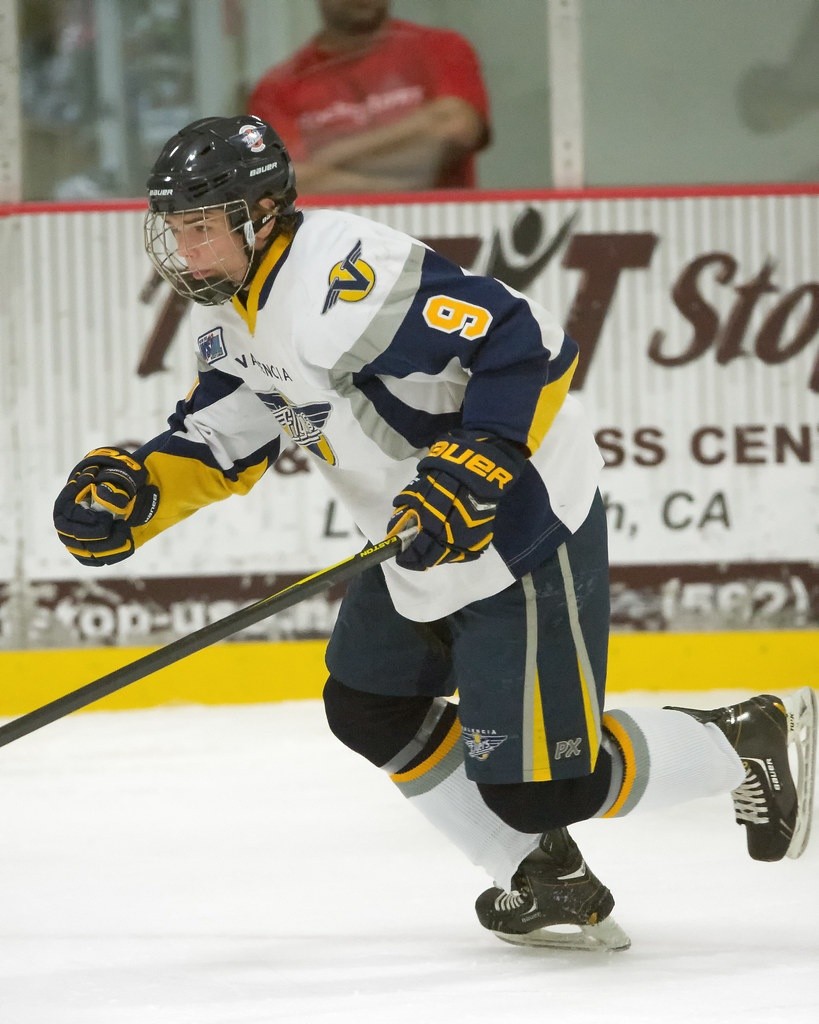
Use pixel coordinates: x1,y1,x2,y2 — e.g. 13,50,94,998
144,113,297,307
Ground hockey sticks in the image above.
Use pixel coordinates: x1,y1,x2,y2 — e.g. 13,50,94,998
1,519,422,744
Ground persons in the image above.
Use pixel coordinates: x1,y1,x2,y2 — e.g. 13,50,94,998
248,0,492,194
53,116,819,953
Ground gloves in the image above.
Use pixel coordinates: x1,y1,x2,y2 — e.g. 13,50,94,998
53,447,161,566
386,425,527,571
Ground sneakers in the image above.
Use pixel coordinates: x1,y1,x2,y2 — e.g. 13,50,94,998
662,687,818,862
475,825,631,952
